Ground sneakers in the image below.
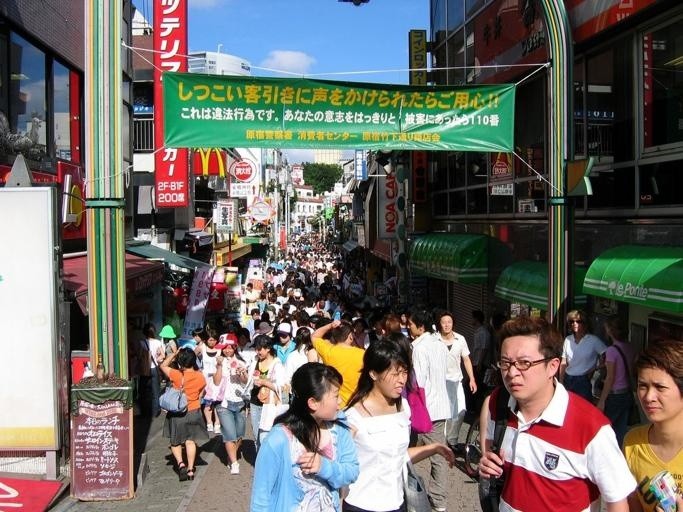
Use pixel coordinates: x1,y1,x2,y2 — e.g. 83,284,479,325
448,441,461,453
226,456,239,475
207,423,221,434
432,506,446,511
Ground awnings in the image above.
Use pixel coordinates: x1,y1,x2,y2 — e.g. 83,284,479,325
408,232,511,283
494,258,589,310
130,244,213,272
582,244,683,313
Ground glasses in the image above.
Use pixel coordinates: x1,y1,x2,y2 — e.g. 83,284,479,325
571,320,582,323
497,358,551,371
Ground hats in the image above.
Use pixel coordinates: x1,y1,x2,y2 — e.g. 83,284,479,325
259,322,272,334
277,323,292,334
159,325,177,339
215,333,237,349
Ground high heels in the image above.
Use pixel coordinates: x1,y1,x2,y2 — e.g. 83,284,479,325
178,462,194,480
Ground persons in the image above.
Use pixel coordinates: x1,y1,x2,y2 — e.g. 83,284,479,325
343,341,455,512
137,320,257,482
436,310,508,457
559,311,637,446
622,337,683,512
249,363,359,512
477,313,639,512
408,310,452,512
239,235,438,452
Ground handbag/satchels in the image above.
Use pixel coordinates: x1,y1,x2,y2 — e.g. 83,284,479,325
407,387,433,433
628,401,640,426
158,386,188,413
260,403,290,431
156,365,163,382
135,350,151,376
404,472,431,512
